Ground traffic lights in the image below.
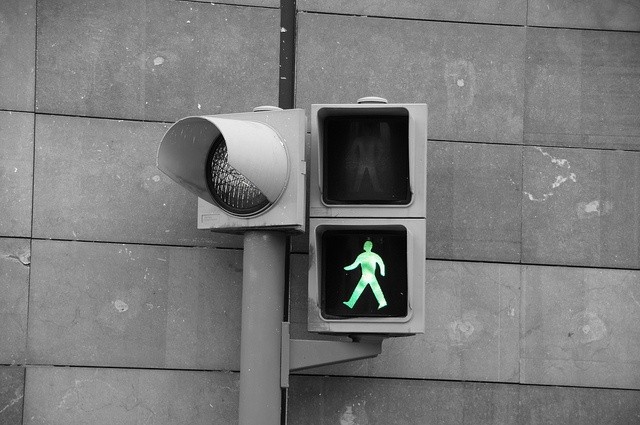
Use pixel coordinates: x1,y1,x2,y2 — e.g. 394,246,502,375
156,104,304,228
307,96,431,335
